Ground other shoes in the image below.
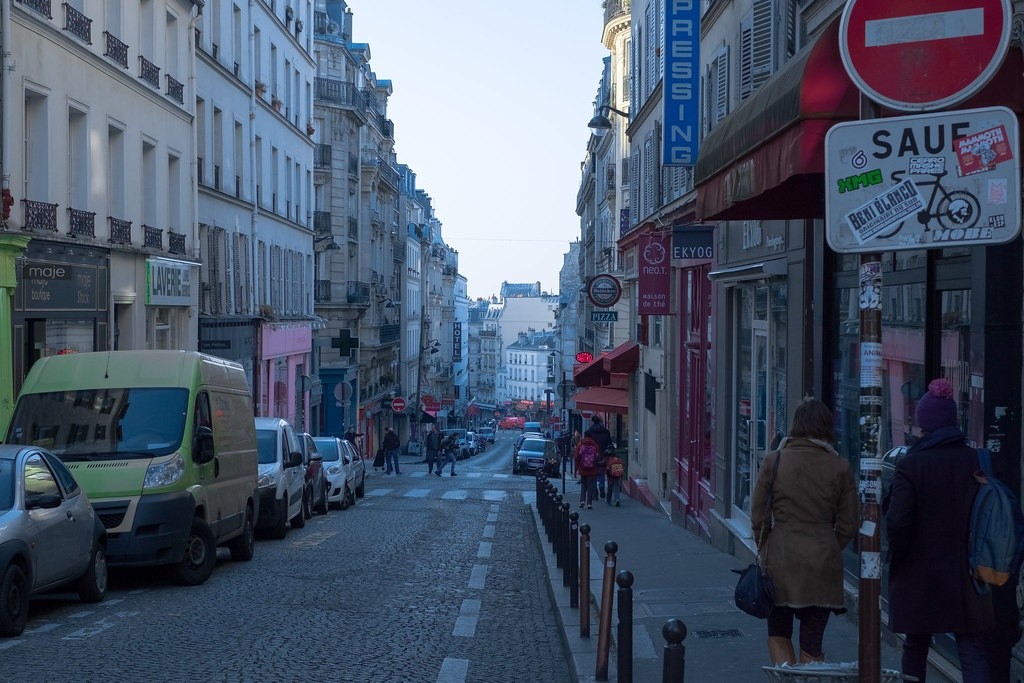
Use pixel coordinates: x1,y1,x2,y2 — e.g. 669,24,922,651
600,487,605,498
605,499,611,505
588,505,592,509
451,473,457,476
592,493,598,500
396,472,402,475
615,499,620,506
387,472,390,475
435,471,441,477
579,502,585,508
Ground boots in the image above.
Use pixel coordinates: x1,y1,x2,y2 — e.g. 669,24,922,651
800,650,824,665
767,635,796,667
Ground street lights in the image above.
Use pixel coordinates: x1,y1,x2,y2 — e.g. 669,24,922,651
413,345,440,440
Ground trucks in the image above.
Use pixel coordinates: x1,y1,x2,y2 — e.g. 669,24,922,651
499,417,524,429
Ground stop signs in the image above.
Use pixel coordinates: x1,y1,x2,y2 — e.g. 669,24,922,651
840,0,1013,113
392,397,406,412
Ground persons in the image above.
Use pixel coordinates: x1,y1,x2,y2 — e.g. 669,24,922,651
603,446,621,506
574,431,601,509
345,426,365,447
435,432,461,478
750,397,860,667
584,416,613,500
425,425,444,475
572,431,581,448
883,379,996,683
382,427,402,475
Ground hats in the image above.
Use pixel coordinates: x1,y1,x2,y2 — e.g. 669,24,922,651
914,378,957,430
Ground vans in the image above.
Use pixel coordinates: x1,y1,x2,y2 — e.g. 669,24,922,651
2,349,261,587
521,422,543,434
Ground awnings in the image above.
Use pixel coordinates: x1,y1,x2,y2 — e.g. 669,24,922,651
571,340,639,415
693,12,1024,223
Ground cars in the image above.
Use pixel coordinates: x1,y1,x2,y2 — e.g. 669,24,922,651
296,432,331,519
0,444,110,638
439,427,498,460
512,432,561,478
253,416,306,535
312,437,366,512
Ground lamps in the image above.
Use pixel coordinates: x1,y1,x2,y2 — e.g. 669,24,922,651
428,339,441,346
422,346,439,354
550,350,561,356
588,106,629,137
379,297,395,308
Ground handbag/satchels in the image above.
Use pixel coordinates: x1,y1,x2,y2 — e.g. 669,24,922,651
730,564,771,620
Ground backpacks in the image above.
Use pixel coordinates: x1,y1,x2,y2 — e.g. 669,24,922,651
578,442,597,469
605,455,624,477
440,436,456,449
968,448,1023,595
